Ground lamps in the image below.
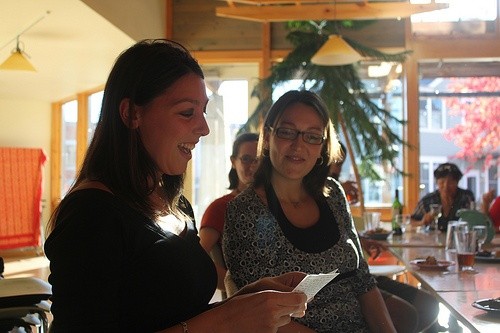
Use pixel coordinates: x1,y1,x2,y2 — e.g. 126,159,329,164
1,35,37,71
310,1,362,66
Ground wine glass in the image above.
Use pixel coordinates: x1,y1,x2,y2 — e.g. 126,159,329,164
430,204,443,235
395,213,411,244
472,225,488,254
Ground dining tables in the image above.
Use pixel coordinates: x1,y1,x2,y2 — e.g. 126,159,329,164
366,221,500,333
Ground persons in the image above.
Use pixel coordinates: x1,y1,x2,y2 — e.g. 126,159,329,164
43,38,311,333
198,89,500,333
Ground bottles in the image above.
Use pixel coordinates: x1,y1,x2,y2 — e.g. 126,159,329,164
392,189,403,236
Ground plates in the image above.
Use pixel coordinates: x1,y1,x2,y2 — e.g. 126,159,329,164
472,298,500,312
474,250,500,261
359,230,392,241
410,258,456,268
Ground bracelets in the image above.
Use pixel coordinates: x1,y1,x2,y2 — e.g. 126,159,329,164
181,321,189,333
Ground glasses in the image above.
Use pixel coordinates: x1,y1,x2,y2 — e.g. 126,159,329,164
270,125,327,146
237,156,260,165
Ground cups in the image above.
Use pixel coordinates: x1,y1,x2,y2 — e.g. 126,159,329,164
454,230,476,273
445,221,469,266
363,212,381,232
